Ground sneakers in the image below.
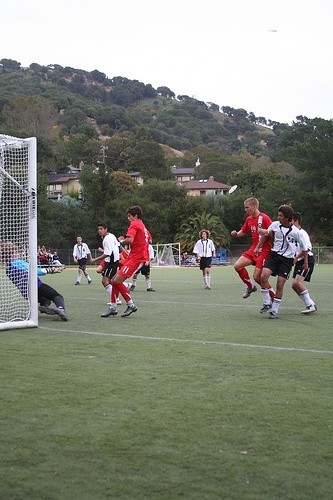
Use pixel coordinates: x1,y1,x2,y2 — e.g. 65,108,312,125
121,304,138,318
269,311,278,319
101,307,118,317
300,304,317,314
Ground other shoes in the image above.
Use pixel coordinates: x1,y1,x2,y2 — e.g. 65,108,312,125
260,304,273,314
55,308,69,321
106,300,122,306
87,279,92,284
243,285,258,298
75,281,80,285
130,285,136,291
127,282,133,292
39,305,58,315
147,287,156,292
204,284,211,290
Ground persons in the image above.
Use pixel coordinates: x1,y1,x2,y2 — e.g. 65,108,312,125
182,251,190,265
72,222,130,306
100,205,153,318
258,204,309,320
291,212,318,315
230,196,276,303
0,240,68,321
129,239,155,292
193,229,217,291
19,242,65,272
